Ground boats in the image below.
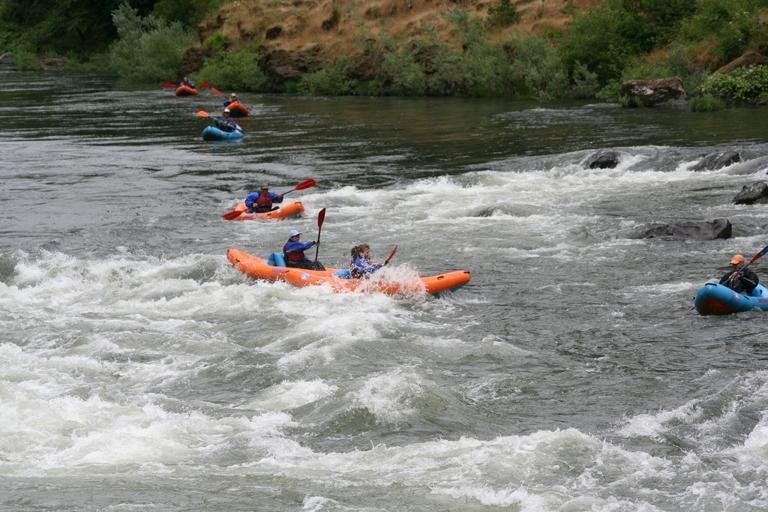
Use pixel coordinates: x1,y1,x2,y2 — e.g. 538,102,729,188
226,200,471,298
694,283,768,315
176,84,250,140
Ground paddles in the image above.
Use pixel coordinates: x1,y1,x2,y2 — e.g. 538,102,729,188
163,83,210,89
223,178,316,220
315,208,325,262
198,111,245,135
211,87,229,100
383,244,398,265
689,246,767,311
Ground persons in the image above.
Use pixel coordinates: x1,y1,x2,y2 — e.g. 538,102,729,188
718,255,760,296
177,76,195,88
216,105,235,133
351,244,383,278
223,93,239,107
282,232,326,271
244,182,284,214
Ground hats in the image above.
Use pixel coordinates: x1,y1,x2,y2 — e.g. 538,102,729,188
728,253,746,265
231,93,237,98
223,108,231,113
288,229,303,240
261,182,269,190
